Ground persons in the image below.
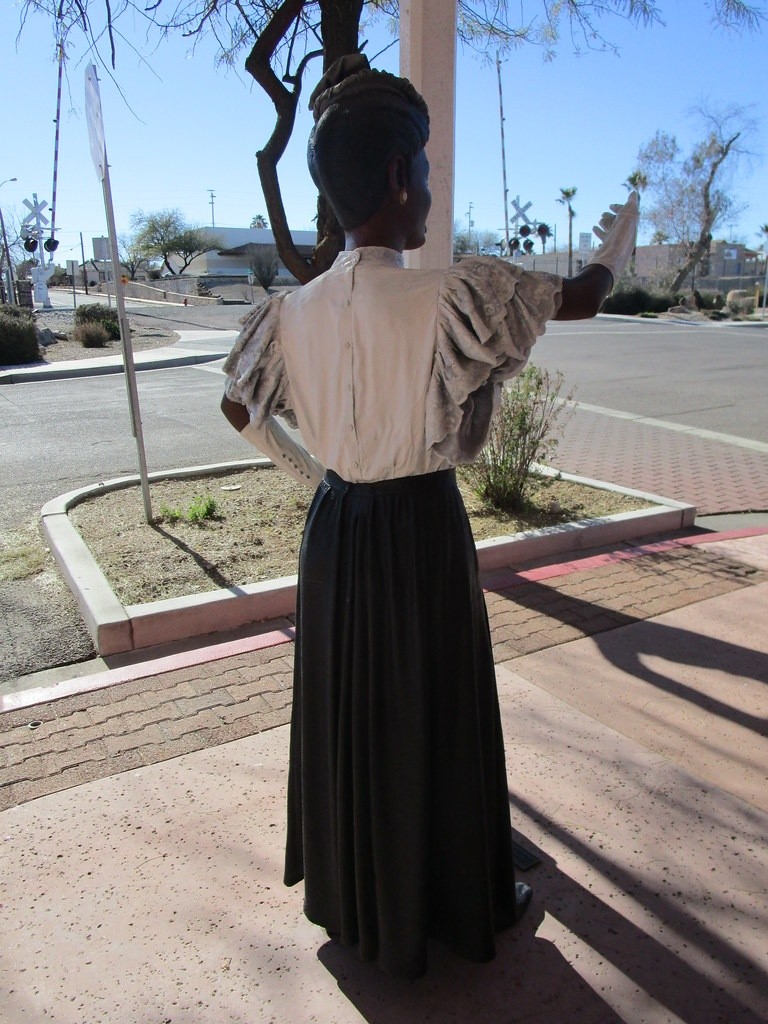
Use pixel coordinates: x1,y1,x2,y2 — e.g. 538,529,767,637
220,52,643,979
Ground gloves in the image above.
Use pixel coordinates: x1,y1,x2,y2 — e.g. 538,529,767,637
580,190,644,294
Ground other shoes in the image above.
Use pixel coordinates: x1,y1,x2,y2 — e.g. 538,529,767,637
514,882,533,917
